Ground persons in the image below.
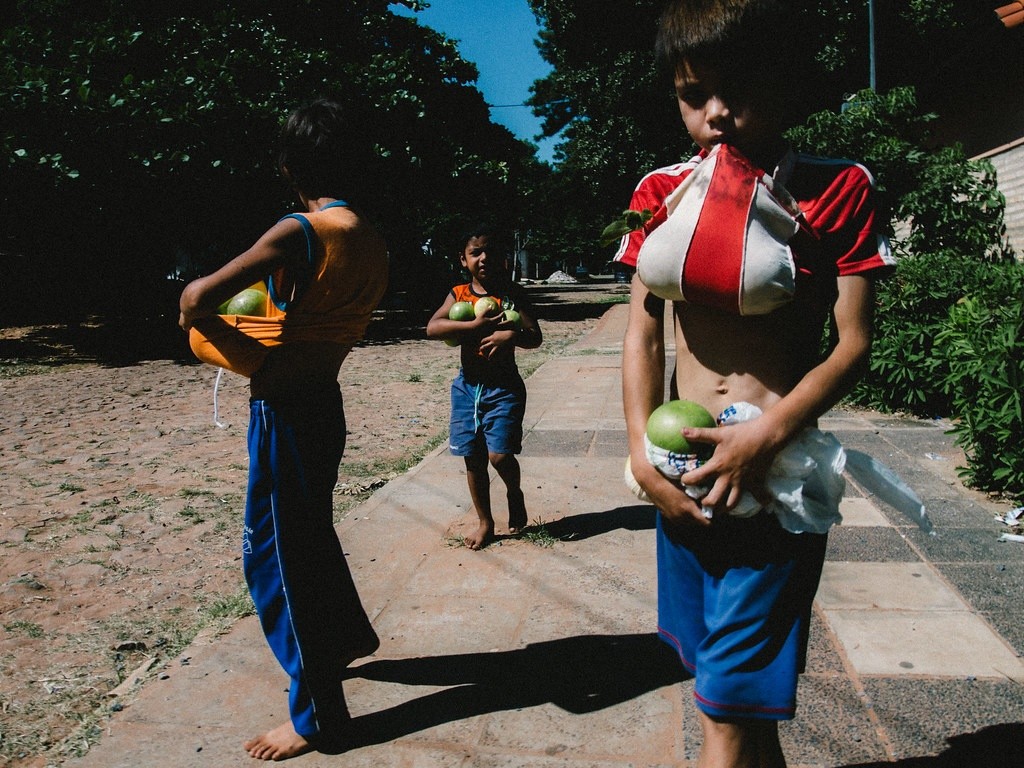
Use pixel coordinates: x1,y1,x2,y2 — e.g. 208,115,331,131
426,223,543,550
178,99,391,761
613,0,898,768
